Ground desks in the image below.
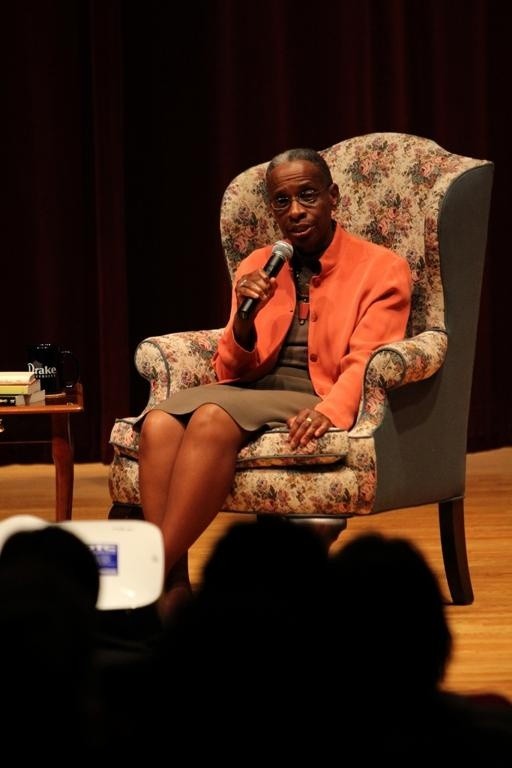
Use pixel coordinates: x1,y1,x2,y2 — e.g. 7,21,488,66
0,383,87,522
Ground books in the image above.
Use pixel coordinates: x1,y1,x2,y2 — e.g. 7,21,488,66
0,371,45,406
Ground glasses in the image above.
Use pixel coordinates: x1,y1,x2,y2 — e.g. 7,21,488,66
271,188,323,211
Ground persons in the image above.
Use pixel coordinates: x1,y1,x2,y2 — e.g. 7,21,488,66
128,145,415,618
0,510,512,768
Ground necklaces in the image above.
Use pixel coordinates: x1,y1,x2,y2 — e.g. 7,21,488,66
295,275,311,325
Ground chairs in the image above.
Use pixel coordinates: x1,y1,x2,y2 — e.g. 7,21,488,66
105,128,496,605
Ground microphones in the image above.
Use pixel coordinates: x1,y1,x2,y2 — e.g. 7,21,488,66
238,240,294,320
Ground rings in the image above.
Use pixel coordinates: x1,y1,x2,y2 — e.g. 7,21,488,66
303,415,313,423
239,279,248,286
